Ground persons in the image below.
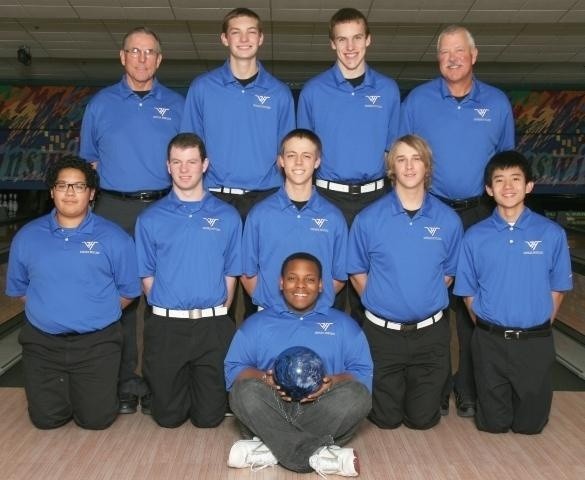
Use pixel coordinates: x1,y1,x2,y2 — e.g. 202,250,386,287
5,154,144,430
180,8,297,417
237,128,349,312
223,252,374,476
450,149,575,435
399,22,516,419
345,133,465,430
294,7,400,325
73,25,186,413
134,132,243,428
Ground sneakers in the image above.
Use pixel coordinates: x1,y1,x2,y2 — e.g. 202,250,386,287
439,394,450,415
141,395,153,415
118,400,137,413
454,393,477,416
309,445,360,477
228,437,278,469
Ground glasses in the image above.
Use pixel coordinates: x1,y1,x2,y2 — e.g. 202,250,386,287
124,48,160,58
55,183,92,192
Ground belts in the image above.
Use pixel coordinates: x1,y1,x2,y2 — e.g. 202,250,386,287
51,332,85,337
99,189,171,200
207,187,250,194
365,309,443,330
316,180,384,196
476,318,551,339
432,195,482,209
153,305,228,319
